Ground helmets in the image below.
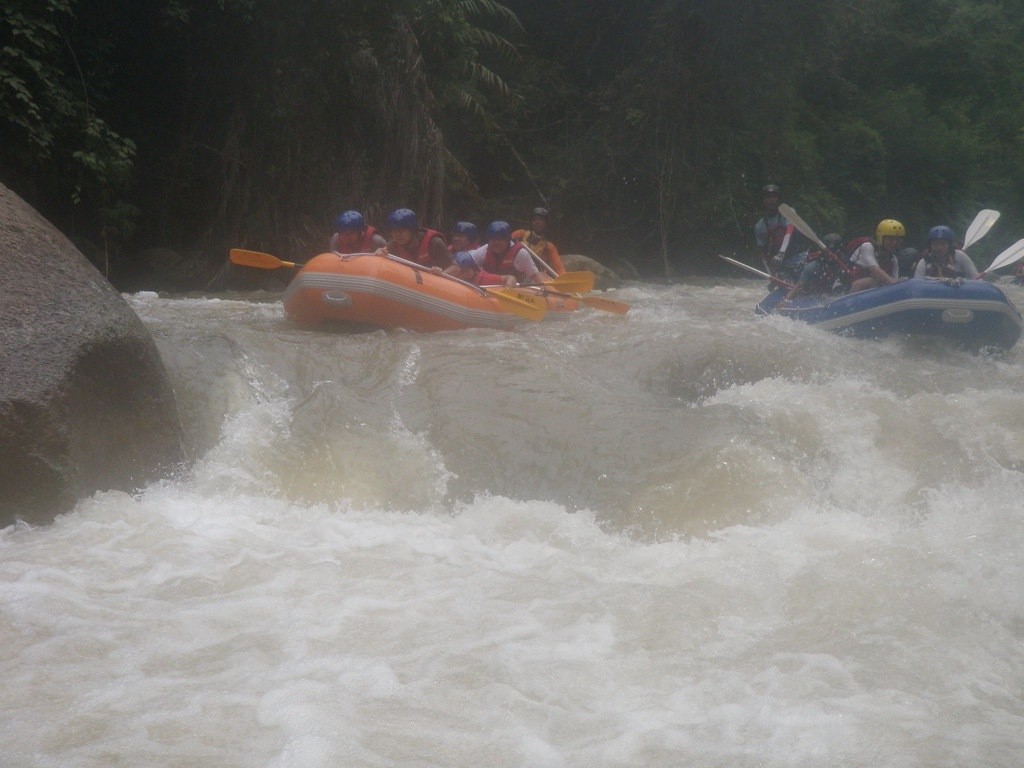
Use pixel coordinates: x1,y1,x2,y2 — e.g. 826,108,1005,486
877,220,906,240
452,222,477,243
899,248,920,269
531,207,552,226
488,220,512,242
824,234,843,252
760,184,778,198
337,210,364,234
387,208,416,234
928,226,955,243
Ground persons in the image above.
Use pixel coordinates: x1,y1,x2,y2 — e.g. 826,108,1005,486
755,185,979,303
331,211,386,254
446,221,481,254
511,207,565,277
431,221,542,290
376,208,459,276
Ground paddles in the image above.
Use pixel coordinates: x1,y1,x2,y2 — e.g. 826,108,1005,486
976,238,1024,280
962,208,1002,251
516,236,583,299
479,271,595,295
228,247,305,270
716,253,794,289
470,282,547,322
777,202,847,271
543,289,632,315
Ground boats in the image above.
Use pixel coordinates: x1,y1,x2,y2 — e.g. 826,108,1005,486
754,276,1024,356
542,289,578,314
283,252,548,334
